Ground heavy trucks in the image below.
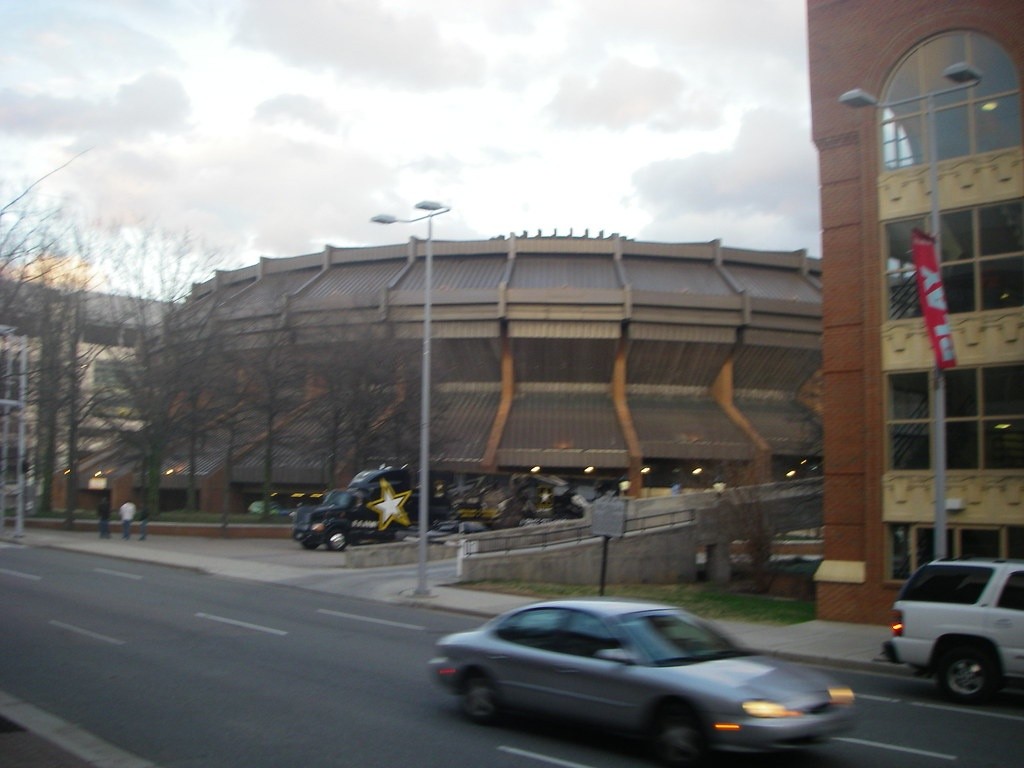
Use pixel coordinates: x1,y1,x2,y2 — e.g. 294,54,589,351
291,463,609,552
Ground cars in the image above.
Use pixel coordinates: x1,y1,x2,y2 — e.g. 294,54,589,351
430,600,856,768
247,498,291,517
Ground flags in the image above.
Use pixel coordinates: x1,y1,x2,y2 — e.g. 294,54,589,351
912,230,958,368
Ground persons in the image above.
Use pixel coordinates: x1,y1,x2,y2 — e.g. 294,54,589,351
96,497,111,538
117,499,137,541
138,498,152,541
671,482,682,495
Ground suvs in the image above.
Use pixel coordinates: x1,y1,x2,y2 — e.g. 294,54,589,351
882,555,1024,707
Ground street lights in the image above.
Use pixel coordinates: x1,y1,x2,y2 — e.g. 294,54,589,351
371,199,453,602
840,63,984,561
